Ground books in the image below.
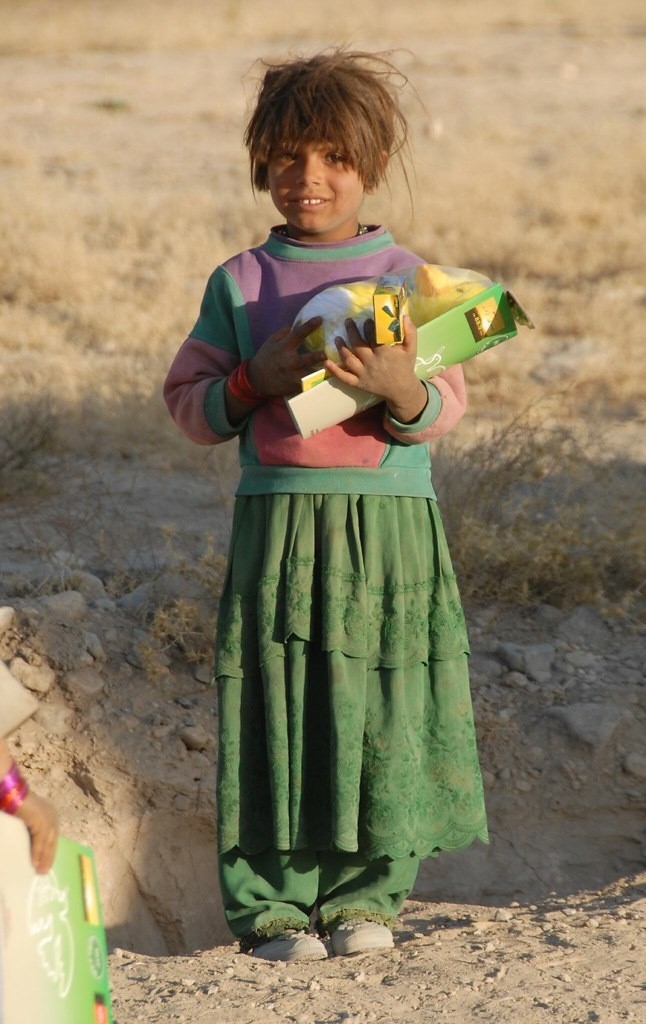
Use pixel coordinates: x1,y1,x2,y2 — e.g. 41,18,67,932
0,812,113,1024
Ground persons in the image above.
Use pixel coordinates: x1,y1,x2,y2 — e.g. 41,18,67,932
164,49,490,964
0,735,60,875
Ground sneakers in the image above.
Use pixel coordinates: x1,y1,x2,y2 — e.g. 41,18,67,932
251,929,326,964
331,918,394,957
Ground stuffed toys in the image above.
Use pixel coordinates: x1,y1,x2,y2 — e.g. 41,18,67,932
288,263,494,389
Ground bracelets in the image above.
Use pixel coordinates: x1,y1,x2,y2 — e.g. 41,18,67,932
0,762,29,815
